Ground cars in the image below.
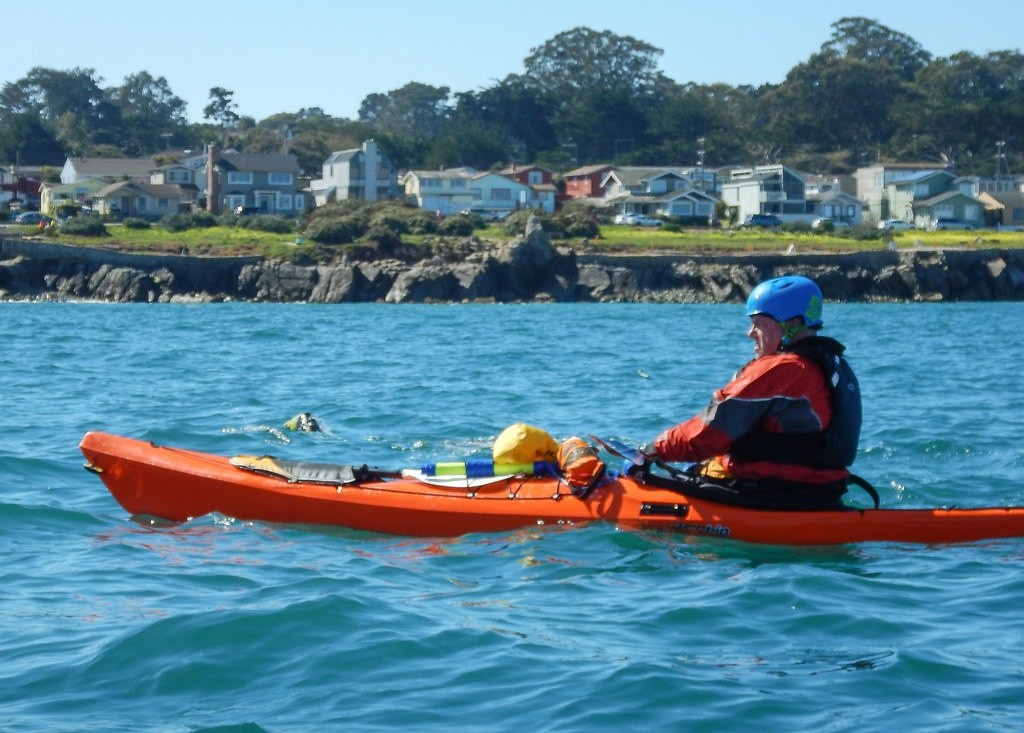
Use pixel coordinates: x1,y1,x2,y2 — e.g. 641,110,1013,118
17,212,54,226
461,206,500,223
747,214,782,227
628,214,666,227
931,216,973,232
879,219,911,231
812,218,849,231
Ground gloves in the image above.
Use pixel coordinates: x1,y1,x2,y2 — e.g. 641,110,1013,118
619,448,654,481
679,459,706,486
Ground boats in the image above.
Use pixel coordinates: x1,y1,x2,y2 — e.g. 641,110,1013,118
78,425,1022,543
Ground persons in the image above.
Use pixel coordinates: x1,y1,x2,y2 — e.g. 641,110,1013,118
639,274,862,504
38,218,57,232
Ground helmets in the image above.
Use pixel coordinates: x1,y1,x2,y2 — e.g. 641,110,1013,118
741,274,825,328
